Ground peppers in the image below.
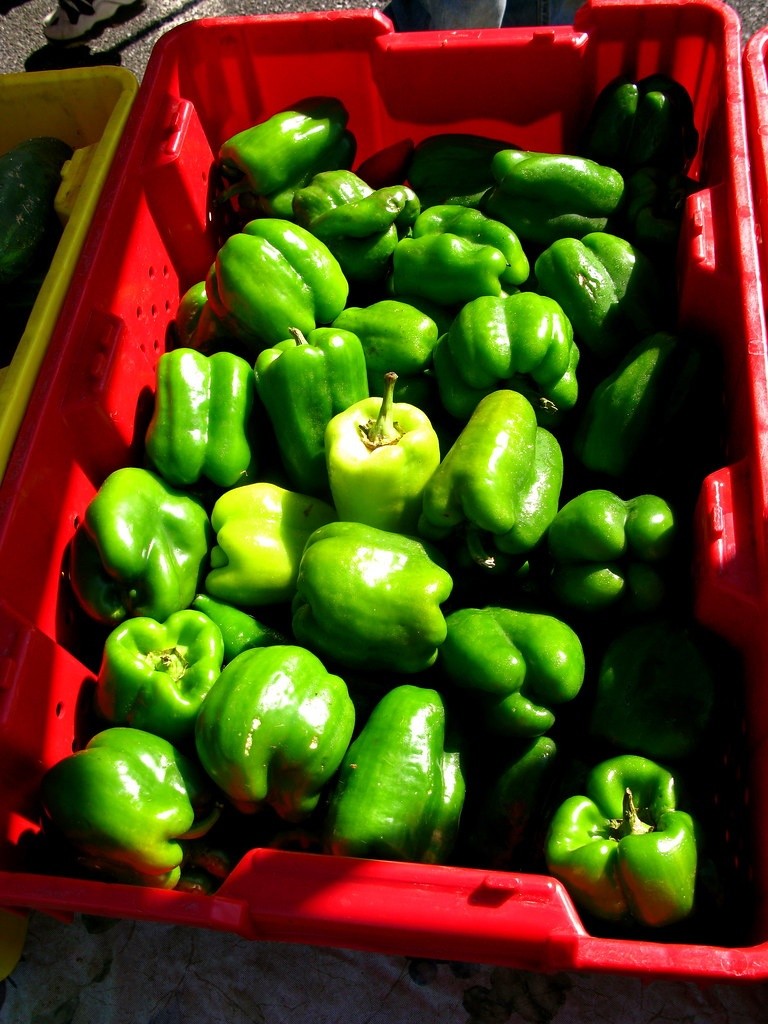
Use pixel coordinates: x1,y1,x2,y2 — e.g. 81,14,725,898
0,73,742,928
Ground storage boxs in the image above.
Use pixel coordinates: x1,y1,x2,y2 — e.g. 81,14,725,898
0,0,768,983
0,65,138,492
742,23,768,321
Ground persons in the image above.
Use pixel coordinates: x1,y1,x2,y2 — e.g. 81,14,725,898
42,0,148,46
419,0,583,28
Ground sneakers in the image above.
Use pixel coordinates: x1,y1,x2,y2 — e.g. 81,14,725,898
40,0,149,46
